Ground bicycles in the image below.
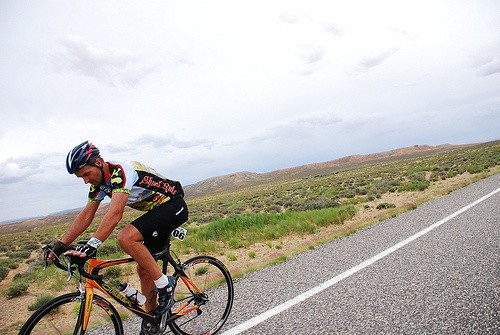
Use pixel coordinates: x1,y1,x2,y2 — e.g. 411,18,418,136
18,216,234,335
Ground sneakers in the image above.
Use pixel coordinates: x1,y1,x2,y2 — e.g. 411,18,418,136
156,276,176,315
139,326,161,335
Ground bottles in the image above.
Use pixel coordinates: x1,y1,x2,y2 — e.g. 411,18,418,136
117,282,146,306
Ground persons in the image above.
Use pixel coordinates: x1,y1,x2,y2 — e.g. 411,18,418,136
42,139,189,335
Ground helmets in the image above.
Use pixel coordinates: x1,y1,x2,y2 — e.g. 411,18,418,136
66,141,99,174
